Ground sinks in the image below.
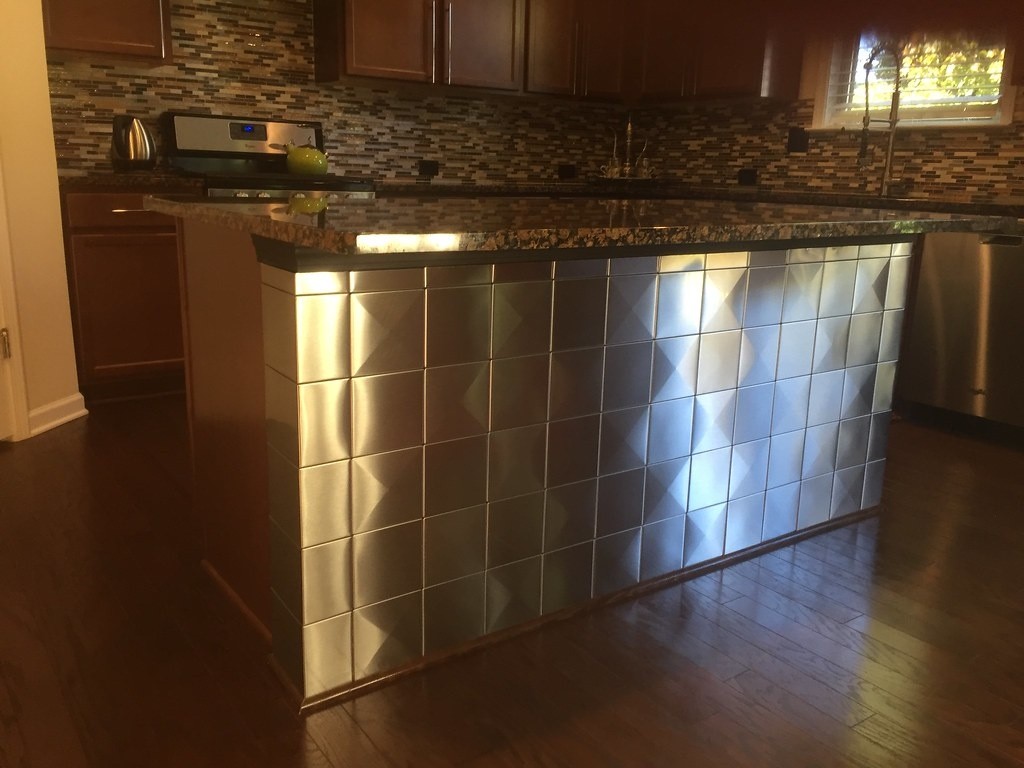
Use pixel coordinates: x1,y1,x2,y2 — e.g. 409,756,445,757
781,193,944,200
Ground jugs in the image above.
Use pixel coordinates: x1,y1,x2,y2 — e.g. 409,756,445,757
284,124,330,175
610,110,648,176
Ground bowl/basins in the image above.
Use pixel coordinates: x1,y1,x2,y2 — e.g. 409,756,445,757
290,198,327,214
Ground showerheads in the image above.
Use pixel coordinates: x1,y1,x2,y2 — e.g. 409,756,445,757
854,127,873,171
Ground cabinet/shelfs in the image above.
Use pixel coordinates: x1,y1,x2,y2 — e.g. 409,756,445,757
900,233,1024,430
313,0,440,88
440,0,524,97
63,191,185,390
524,0,816,101
40,0,172,68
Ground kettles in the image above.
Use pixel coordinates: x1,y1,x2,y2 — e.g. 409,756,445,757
112,114,157,170
